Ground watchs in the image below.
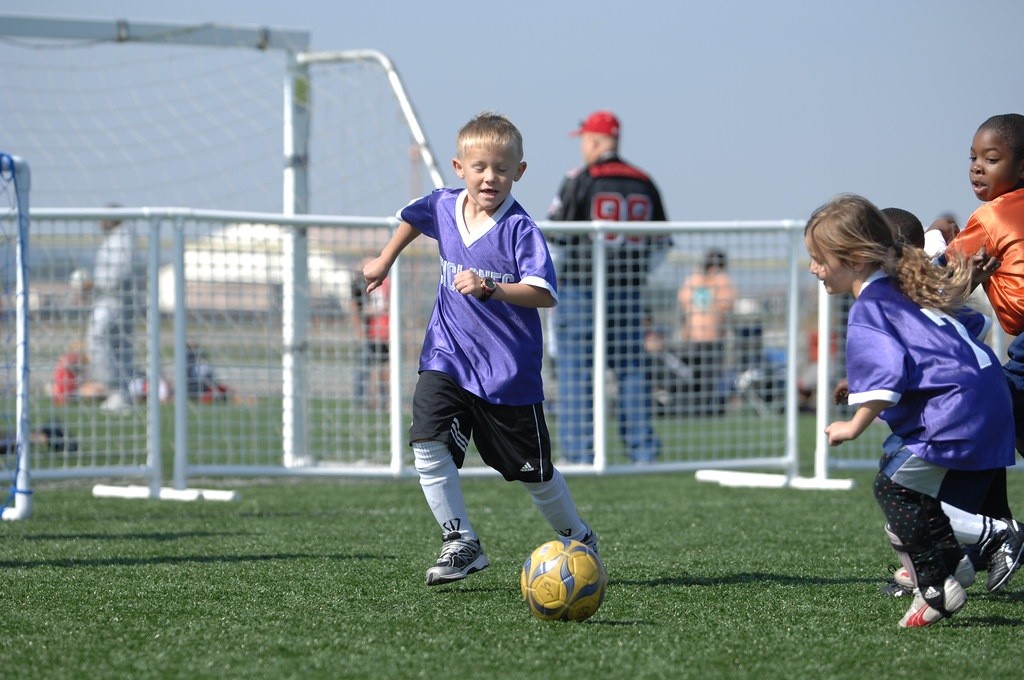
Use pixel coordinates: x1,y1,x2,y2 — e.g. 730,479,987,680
476,275,497,303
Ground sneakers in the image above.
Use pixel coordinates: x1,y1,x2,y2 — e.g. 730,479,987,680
422,530,489,585
894,574,966,631
889,555,975,588
981,515,1024,591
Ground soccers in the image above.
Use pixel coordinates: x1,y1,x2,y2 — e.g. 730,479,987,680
520,538,608,622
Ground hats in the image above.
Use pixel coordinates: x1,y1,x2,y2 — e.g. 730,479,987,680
568,109,621,138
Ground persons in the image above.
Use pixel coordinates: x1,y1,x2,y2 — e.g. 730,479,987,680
362,113,599,587
51,200,235,414
349,255,390,400
919,113,1024,463
541,108,675,468
675,246,740,417
803,194,1024,631
643,304,788,417
